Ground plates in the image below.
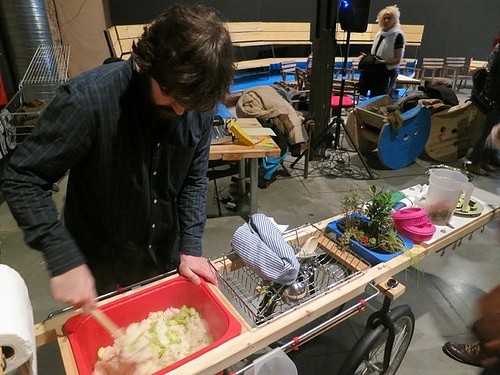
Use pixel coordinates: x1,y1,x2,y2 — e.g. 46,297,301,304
454,194,484,215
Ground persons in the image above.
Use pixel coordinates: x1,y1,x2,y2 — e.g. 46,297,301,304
361,4,406,94
466,35,500,175
0,2,238,312
443,319,495,369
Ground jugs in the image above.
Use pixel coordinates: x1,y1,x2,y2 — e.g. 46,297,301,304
425,168,473,226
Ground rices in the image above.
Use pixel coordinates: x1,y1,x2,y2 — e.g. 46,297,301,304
92,306,216,375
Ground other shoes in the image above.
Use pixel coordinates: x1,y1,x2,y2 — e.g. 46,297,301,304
483,164,496,172
468,163,489,175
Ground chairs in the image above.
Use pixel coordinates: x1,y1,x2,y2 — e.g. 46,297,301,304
279,56,488,109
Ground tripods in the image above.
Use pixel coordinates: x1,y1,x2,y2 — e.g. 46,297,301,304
290,30,375,180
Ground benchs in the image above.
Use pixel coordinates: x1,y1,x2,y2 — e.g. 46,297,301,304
105,22,425,68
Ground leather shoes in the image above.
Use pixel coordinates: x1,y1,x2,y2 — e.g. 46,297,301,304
473,312,500,341
442,339,500,368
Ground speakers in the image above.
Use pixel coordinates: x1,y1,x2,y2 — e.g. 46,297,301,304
340,0,371,32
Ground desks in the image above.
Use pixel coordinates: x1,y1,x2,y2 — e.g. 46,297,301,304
208,118,280,218
396,72,420,97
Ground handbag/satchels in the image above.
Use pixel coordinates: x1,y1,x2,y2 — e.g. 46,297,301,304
470,69,497,116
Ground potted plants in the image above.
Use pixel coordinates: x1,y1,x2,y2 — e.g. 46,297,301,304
327,185,412,263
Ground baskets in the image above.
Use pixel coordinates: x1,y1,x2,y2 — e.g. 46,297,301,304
209,222,372,329
